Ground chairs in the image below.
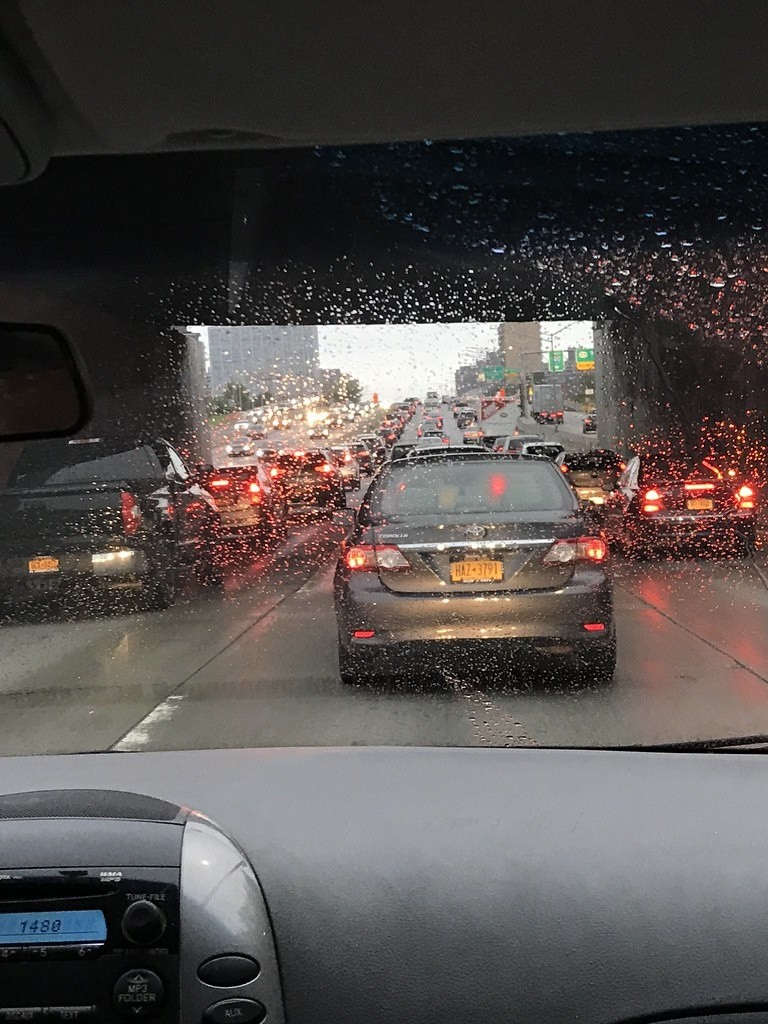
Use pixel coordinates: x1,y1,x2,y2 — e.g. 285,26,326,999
397,489,441,514
499,484,547,509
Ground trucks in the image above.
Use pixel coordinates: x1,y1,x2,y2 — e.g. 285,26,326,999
529,384,566,425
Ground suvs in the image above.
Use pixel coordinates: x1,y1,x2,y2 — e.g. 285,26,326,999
0,430,221,610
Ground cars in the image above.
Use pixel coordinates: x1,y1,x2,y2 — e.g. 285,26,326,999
224,396,571,522
580,412,597,433
327,452,618,688
554,448,627,508
204,461,287,541
602,453,757,562
